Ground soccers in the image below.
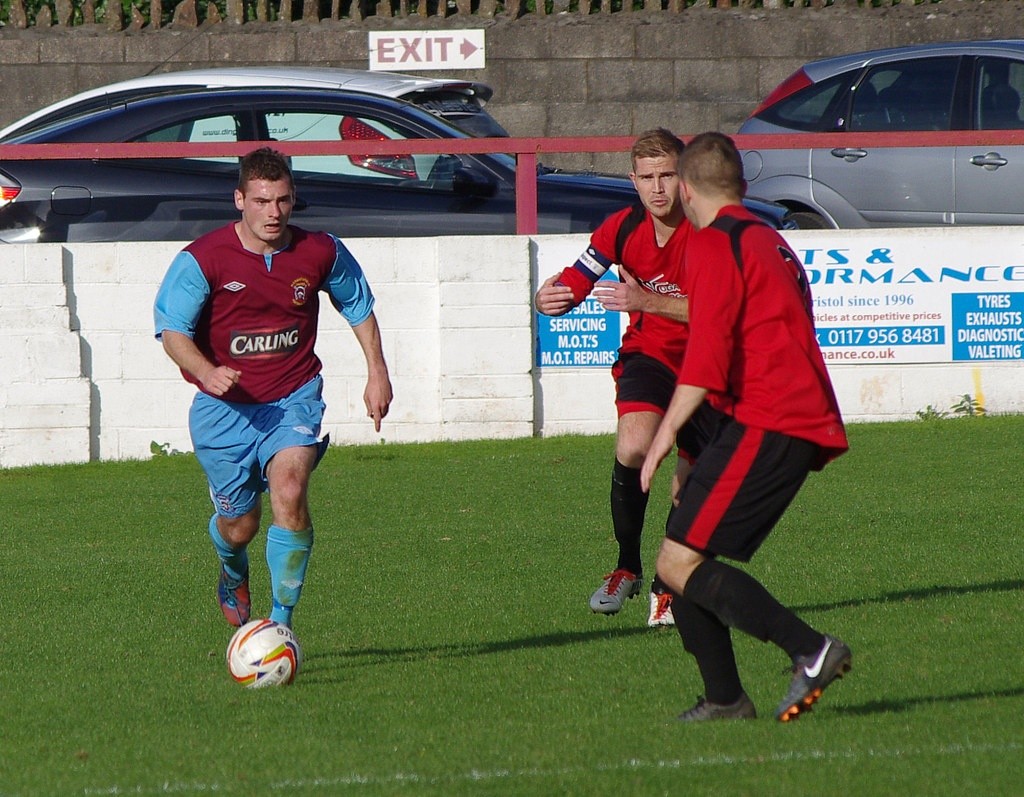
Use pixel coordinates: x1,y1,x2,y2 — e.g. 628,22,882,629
226,619,300,689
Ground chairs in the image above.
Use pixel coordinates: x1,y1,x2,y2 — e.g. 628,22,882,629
820,80,876,128
982,83,1022,127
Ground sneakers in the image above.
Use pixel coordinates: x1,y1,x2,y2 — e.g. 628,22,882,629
217,560,251,627
649,592,676,628
678,690,757,721
776,633,852,723
590,572,643,615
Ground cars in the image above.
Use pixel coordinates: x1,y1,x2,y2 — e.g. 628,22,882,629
0,65,514,183
0,85,802,244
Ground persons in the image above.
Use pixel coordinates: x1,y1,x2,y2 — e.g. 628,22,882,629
152,147,393,636
637,128,853,726
535,129,722,628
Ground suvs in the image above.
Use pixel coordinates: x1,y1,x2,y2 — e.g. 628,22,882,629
732,38,1024,231
